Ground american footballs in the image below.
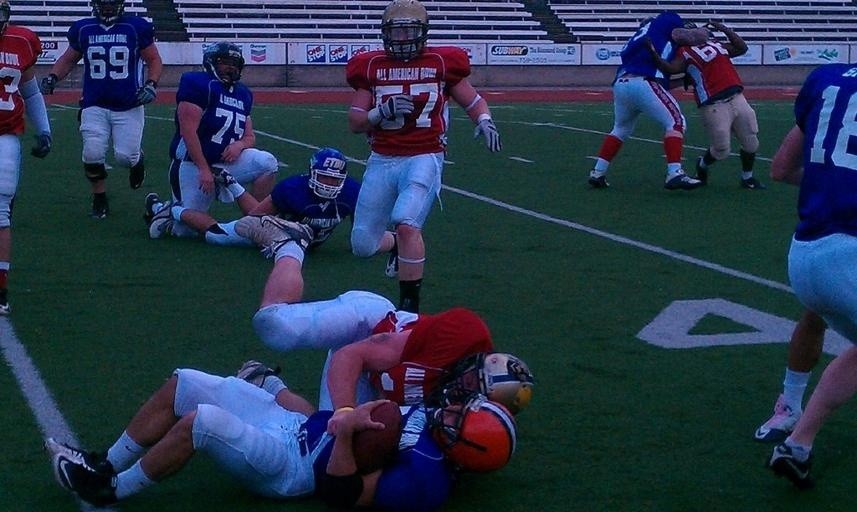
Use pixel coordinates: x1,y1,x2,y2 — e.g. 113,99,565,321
351,401,402,475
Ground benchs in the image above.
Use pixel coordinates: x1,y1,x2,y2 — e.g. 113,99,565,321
172,2,553,45
9,0,156,41
548,2,856,45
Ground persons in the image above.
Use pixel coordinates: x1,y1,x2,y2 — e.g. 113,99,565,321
345,2,503,314
589,10,764,190
144,40,363,245
36,2,162,216
0,0,53,316
45,216,533,511
753,62,857,493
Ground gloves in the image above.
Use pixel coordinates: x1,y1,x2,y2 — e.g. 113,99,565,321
472,114,501,152
134,78,158,107
30,134,52,157
40,72,60,98
367,94,415,126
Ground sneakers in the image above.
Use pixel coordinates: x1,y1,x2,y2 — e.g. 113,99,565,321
92,196,109,219
384,231,400,277
144,192,182,240
754,411,801,440
588,170,611,188
765,443,816,493
42,435,120,511
234,214,314,257
694,155,708,187
663,168,701,190
128,149,145,190
739,174,767,192
207,163,236,189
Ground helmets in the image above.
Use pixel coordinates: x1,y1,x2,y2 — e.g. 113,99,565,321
1,1,12,38
87,1,124,24
308,148,348,200
202,40,244,84
425,350,534,474
377,0,430,61
